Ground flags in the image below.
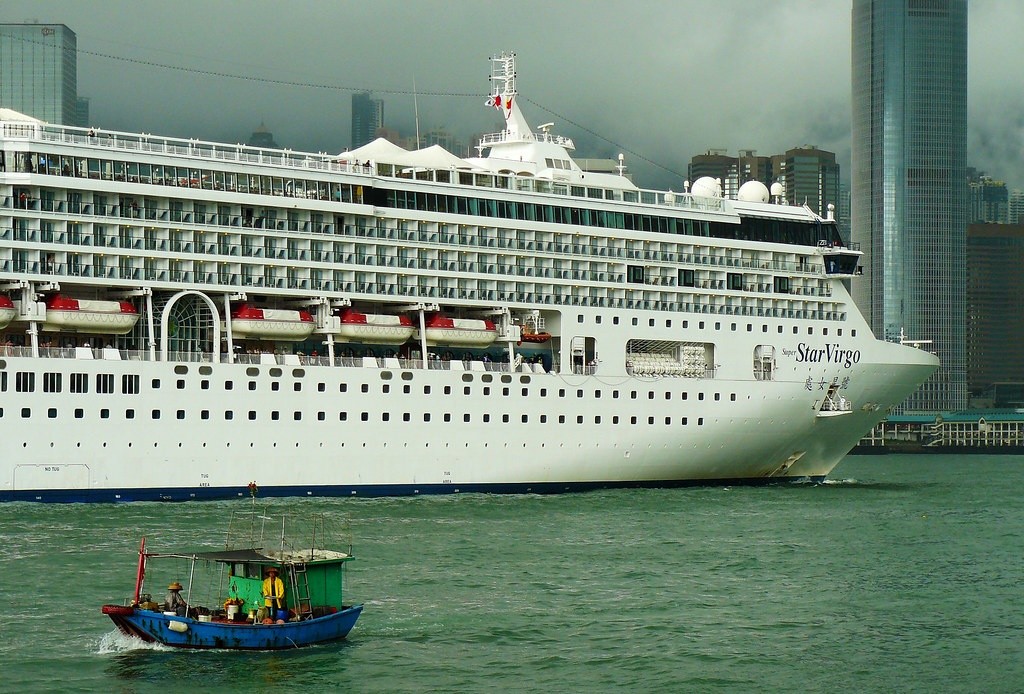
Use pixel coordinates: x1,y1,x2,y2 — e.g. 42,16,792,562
507,98,513,109
496,96,501,105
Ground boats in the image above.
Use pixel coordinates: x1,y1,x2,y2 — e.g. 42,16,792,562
41,293,141,335
0,294,19,329
102,536,364,650
333,308,415,345
425,313,499,348
231,302,316,341
521,332,552,343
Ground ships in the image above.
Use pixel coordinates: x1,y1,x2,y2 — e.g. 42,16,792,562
0,49,941,505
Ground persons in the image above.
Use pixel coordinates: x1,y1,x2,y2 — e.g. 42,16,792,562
262,568,284,620
353,159,370,167
105,343,112,348
428,354,450,361
234,347,269,354
311,350,318,356
840,396,845,409
829,397,833,410
274,348,289,355
460,353,491,362
340,352,345,356
6,340,14,346
165,583,187,612
394,352,406,359
297,350,303,355
84,341,91,347
39,340,52,347
197,345,202,351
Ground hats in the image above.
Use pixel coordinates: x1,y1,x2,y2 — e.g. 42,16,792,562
265,567,279,577
168,582,183,590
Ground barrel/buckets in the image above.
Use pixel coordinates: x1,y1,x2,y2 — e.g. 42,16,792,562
198,615,212,623
227,605,239,620
163,612,177,617
247,607,269,624
